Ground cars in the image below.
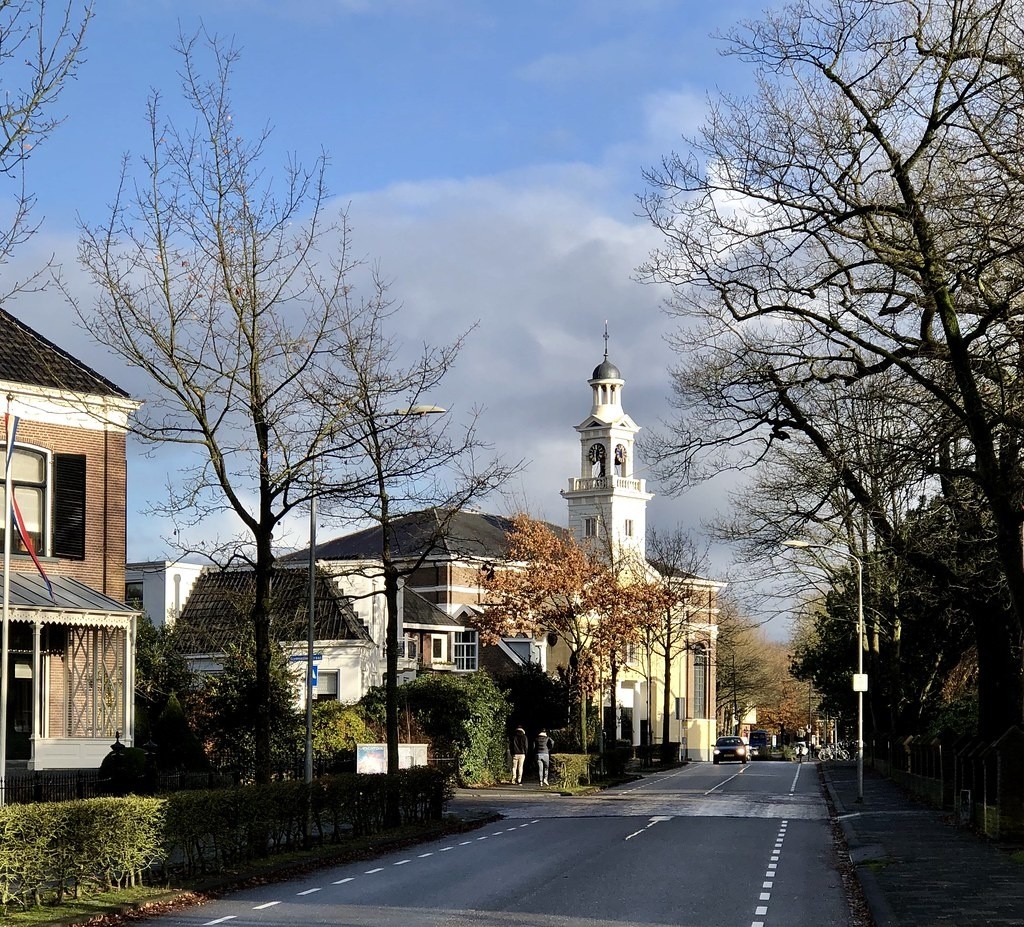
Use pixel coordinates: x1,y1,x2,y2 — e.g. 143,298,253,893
711,736,750,763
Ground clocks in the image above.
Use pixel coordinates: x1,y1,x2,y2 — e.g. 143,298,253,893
615,444,628,463
588,443,605,463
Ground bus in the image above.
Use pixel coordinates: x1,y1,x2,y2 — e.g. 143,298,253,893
749,729,772,750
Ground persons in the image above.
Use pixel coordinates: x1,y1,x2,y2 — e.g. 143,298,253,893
510,725,555,787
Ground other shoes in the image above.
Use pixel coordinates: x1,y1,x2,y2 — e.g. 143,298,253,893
517,780,522,785
511,780,516,784
540,782,543,786
544,779,549,785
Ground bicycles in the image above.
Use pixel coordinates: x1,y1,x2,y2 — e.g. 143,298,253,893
818,741,850,761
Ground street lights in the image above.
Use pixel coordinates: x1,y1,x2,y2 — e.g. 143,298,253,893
305,405,447,779
780,539,869,800
646,628,691,767
